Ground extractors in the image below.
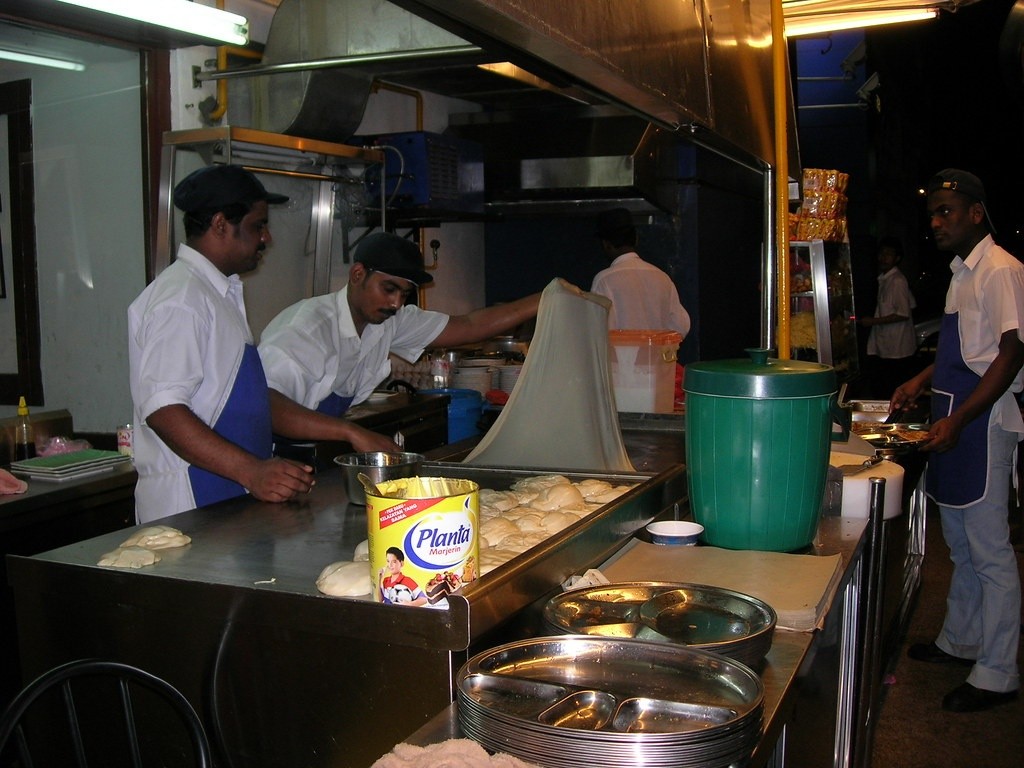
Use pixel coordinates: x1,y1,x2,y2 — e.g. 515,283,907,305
193,0,507,137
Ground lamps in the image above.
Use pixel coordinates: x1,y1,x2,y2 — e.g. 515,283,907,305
59,0,250,46
0,39,87,71
784,6,940,38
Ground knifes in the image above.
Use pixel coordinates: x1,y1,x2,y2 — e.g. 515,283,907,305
837,455,884,476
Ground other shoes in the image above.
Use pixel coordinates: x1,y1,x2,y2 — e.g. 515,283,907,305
942,680,1019,712
909,642,961,664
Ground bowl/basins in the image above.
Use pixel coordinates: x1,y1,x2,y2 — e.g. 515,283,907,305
334,450,426,505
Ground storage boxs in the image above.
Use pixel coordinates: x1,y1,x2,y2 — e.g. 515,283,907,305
608,327,682,414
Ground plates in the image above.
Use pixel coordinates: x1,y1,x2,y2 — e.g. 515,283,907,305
456,581,777,768
9,447,130,482
450,358,526,397
363,389,400,403
859,423,932,449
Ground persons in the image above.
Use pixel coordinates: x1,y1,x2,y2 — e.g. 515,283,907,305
259,231,544,468
865,236,919,400
591,207,690,411
127,165,315,526
889,169,1024,712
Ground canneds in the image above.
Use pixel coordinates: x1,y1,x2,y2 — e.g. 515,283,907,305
116,424,134,458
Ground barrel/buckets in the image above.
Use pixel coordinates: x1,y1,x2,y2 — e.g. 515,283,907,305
680,348,838,553
364,477,481,609
605,327,683,414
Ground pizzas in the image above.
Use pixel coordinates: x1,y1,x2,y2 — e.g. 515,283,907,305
890,430,930,441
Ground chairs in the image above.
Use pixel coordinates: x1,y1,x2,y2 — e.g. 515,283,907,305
206,599,280,768
0,660,214,768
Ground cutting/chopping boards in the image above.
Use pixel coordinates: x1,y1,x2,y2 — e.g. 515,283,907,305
826,450,904,520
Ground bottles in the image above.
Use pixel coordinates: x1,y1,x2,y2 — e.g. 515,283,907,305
428,350,449,389
116,423,134,459
14,396,37,461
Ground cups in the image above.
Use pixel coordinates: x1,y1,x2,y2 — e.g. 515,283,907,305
646,520,704,550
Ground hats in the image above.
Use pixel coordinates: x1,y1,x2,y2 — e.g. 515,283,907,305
354,232,433,287
172,164,290,215
929,169,998,236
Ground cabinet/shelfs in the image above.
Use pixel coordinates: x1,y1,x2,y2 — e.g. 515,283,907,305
789,239,859,386
